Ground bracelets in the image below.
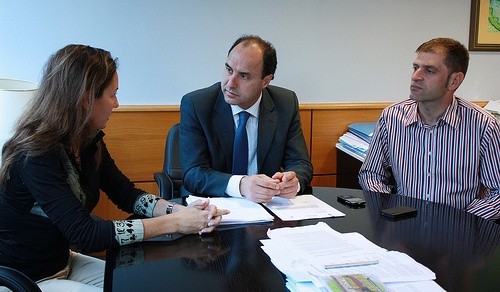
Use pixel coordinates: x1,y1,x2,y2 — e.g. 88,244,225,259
166,201,176,214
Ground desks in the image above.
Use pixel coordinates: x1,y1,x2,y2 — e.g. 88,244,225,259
335,149,396,193
102,186,500,292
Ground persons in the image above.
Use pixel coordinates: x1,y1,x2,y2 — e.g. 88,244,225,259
0,44,230,292
358,38,500,221
179,35,313,203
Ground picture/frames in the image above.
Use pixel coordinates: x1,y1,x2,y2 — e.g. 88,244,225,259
468,0,500,52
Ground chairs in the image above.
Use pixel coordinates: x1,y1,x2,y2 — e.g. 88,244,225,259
153,123,181,200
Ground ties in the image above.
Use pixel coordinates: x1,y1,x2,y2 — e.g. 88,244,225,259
232,112,251,175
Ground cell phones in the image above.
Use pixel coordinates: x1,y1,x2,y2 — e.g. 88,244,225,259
336,195,366,206
380,207,417,219
